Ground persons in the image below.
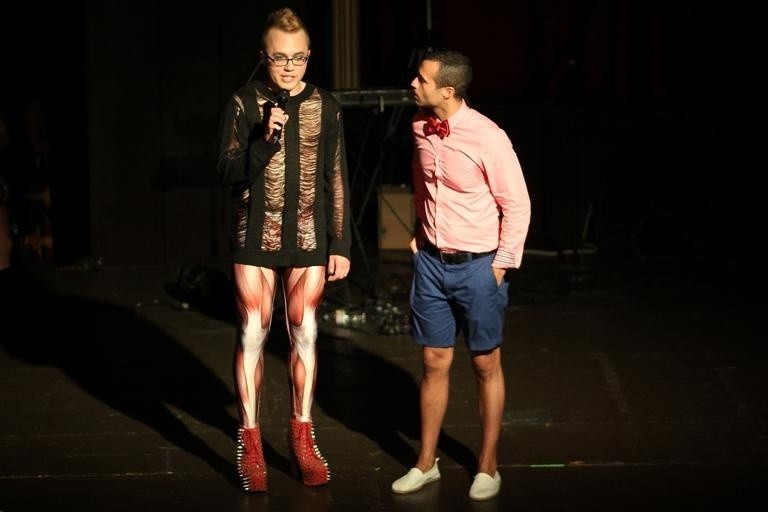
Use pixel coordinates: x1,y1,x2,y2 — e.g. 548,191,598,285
213,6,355,497
387,46,534,504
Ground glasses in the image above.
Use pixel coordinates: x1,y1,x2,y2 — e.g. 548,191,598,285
263,49,310,67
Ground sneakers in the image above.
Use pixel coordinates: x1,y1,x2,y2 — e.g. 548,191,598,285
468,468,502,501
391,457,442,494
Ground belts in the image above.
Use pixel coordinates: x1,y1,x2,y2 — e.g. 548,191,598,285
421,237,499,265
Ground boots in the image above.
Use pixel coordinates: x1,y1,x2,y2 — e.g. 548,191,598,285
236,421,268,492
287,416,330,486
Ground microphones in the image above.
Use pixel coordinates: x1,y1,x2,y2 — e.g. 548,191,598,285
272,89,289,140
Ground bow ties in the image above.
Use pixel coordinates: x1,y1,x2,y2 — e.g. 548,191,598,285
423,116,450,140
